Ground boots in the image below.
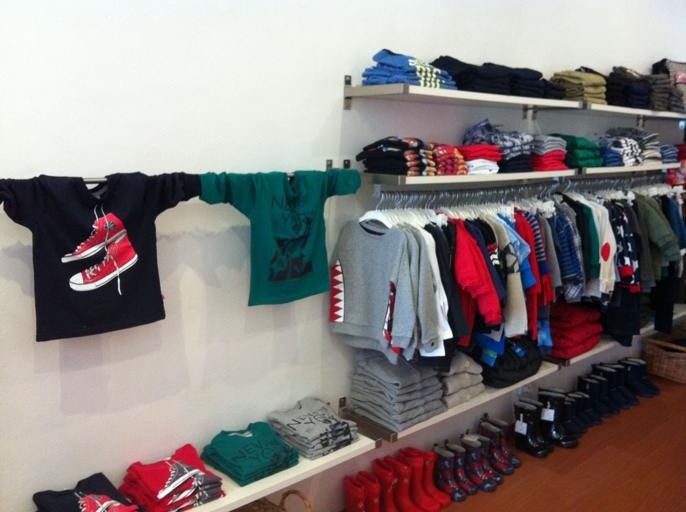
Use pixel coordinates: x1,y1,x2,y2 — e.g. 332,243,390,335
513,358,660,458
343,413,522,512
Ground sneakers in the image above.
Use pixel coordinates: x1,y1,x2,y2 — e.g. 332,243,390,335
61,203,127,263
69,208,138,295
73,491,139,512
152,460,201,500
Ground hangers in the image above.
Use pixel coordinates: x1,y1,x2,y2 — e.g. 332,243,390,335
359,173,673,229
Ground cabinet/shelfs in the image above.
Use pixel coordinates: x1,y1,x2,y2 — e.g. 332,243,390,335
534,102,686,367
339,74,583,440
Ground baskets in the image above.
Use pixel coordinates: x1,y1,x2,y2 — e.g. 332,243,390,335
644,323,686,383
